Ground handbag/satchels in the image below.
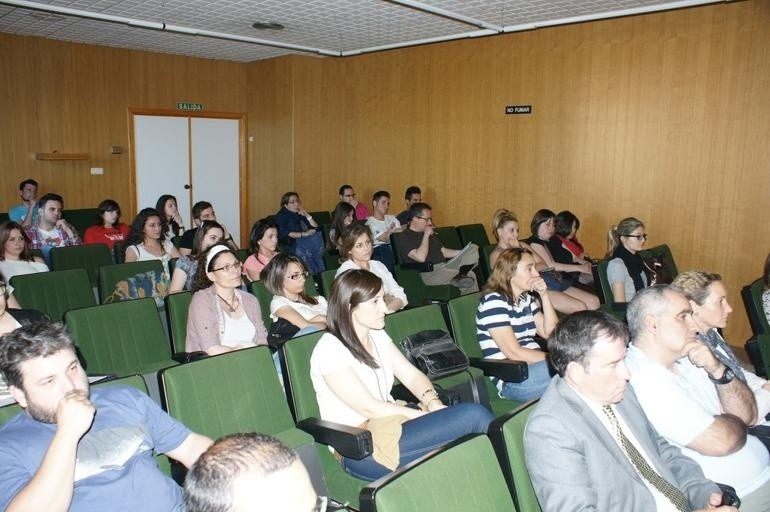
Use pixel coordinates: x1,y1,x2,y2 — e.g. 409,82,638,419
110,270,172,310
401,329,470,380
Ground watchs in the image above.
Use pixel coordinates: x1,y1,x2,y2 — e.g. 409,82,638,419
707,364,735,385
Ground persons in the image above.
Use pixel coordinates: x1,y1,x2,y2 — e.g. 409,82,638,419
0,221,50,280
309,268,496,483
124,194,239,293
185,243,266,357
0,272,44,335
184,433,330,512
8,179,40,228
624,283,770,512
23,193,82,271
243,184,476,334
84,199,133,264
474,209,662,401
670,271,770,429
0,321,214,512
522,310,738,512
762,253,770,327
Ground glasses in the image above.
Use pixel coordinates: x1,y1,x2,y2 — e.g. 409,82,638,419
288,200,301,204
212,262,241,273
0,284,8,295
415,216,432,224
621,234,649,242
343,194,356,198
284,274,307,281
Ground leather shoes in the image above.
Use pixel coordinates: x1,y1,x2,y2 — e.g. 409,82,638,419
452,277,474,289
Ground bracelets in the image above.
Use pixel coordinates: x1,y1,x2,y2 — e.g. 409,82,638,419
427,399,440,409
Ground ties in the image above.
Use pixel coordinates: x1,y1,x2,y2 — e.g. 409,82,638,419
601,404,693,512
